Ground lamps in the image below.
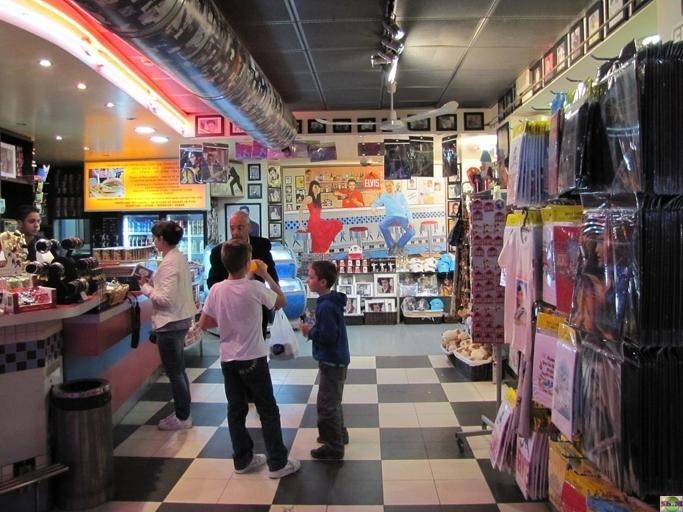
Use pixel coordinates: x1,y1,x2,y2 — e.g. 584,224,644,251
381,13,405,40
381,35,404,54
377,46,399,62
371,55,391,67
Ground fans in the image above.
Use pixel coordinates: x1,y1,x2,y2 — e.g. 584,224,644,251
314,79,459,130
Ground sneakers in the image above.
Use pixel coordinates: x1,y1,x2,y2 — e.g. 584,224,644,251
233,454,267,474
158,415,192,430
317,436,348,444
160,411,175,423
269,458,301,478
311,445,344,458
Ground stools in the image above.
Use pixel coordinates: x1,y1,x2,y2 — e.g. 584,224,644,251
421,220,438,258
295,229,312,253
350,226,367,249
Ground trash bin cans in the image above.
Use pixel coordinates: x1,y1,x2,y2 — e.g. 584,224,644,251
51,378,111,512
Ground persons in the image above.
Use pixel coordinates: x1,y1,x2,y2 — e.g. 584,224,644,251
16,205,83,262
137,220,199,430
305,169,312,194
200,120,217,133
268,167,279,180
596,233,612,294
185,152,203,174
207,212,279,342
439,276,453,295
333,179,365,208
299,261,350,461
377,278,394,293
298,180,343,254
370,180,416,255
201,153,223,183
240,206,259,236
198,238,301,478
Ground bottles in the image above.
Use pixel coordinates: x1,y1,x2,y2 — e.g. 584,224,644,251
250,260,268,272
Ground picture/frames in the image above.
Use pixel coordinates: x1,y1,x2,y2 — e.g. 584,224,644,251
447,184,461,200
448,201,460,217
605,0,628,35
568,18,584,66
295,175,304,209
631,0,646,14
355,282,373,297
286,204,293,210
343,295,361,315
407,114,430,131
436,114,457,131
286,195,292,203
381,118,400,132
339,274,355,285
285,176,292,183
268,222,282,240
357,117,376,132
333,118,352,133
496,121,510,161
543,34,567,87
585,0,603,51
373,274,396,296
308,119,326,133
464,112,484,130
268,204,282,221
296,120,302,133
267,187,281,203
497,96,505,122
384,298,395,311
285,185,292,193
0,141,16,179
230,122,247,135
449,219,457,251
448,163,461,183
194,114,224,137
530,59,542,96
407,177,417,189
248,163,261,181
505,88,513,116
365,300,384,312
337,285,353,295
248,183,262,199
281,163,447,263
224,202,261,241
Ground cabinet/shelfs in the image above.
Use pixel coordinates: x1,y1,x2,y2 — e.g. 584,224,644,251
0,127,34,184
64,293,164,430
0,291,108,484
284,205,445,248
318,180,375,195
